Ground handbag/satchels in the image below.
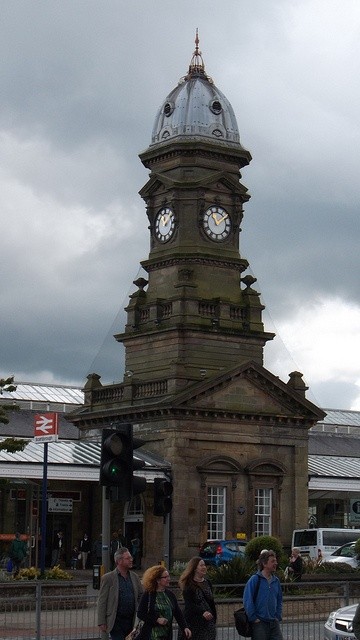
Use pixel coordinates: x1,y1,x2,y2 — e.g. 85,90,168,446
125,620,144,639
234,608,249,637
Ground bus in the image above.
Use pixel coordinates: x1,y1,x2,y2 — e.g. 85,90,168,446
290,526,360,566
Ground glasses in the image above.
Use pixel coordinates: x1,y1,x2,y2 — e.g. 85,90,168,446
161,575,170,580
123,556,133,560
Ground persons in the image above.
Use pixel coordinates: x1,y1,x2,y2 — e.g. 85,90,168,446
78,531,91,570
51,530,63,570
351,538,360,640
98,547,144,640
137,565,191,639
242,548,284,639
70,545,79,571
286,546,304,582
110,533,122,566
9,533,28,577
94,533,103,563
116,528,128,548
179,556,218,639
131,532,142,567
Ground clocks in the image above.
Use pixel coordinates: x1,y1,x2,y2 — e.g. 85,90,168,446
202,203,232,242
153,206,176,244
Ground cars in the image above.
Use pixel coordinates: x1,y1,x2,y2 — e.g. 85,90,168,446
317,541,360,568
198,539,249,567
323,603,360,640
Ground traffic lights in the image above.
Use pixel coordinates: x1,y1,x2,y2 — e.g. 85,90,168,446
110,421,147,503
153,477,173,517
100,428,128,488
